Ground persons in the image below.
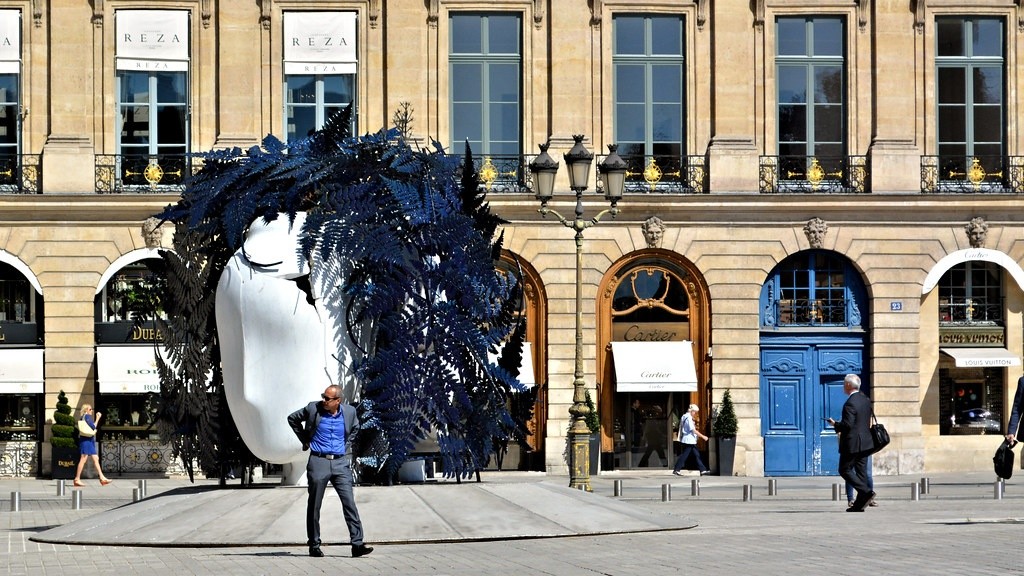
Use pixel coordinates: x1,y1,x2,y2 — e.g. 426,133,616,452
802,216,828,248
641,216,666,245
631,399,646,466
287,385,374,556
638,405,668,467
140,217,163,247
1005,376,1024,446
73,403,112,487
829,374,878,512
964,216,988,246
672,403,710,476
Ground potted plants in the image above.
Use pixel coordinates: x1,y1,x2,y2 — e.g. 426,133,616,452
567,387,601,475
49,390,81,480
713,387,739,476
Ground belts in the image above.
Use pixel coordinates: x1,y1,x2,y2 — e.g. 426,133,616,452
311,451,343,460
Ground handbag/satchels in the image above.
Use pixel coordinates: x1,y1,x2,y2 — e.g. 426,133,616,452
992,436,1018,479
77,414,95,437
869,408,890,453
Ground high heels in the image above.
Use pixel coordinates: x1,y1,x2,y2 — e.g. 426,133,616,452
74,478,86,486
100,478,113,486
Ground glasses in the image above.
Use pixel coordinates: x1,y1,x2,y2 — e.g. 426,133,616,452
321,393,340,401
89,409,93,411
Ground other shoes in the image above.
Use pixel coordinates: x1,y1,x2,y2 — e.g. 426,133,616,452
700,471,710,477
673,471,684,477
309,548,324,557
351,545,374,557
848,500,855,507
869,501,878,507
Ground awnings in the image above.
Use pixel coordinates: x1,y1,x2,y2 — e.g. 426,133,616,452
363,341,535,392
0,349,45,393
609,341,698,392
940,348,1022,367
97,346,224,393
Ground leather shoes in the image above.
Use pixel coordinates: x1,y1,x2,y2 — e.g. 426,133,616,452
860,491,876,510
846,506,864,512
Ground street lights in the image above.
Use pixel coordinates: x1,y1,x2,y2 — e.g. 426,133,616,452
528,135,630,494
